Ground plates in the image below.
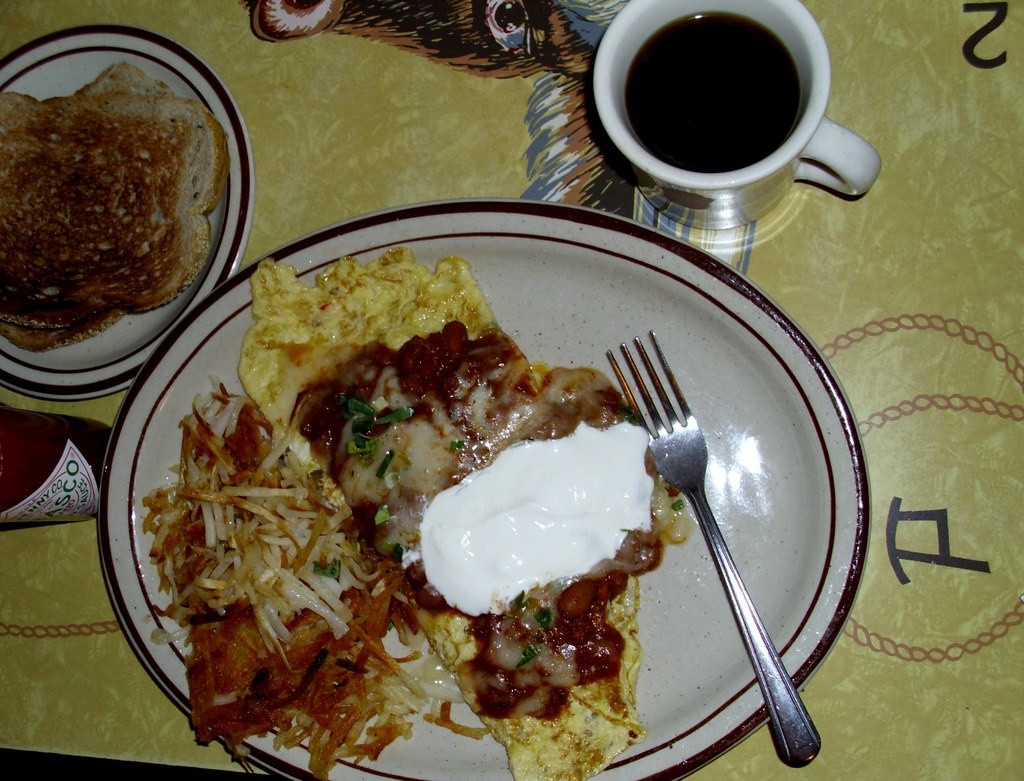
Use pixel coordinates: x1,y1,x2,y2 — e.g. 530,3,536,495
0,24,255,402
96,196,870,781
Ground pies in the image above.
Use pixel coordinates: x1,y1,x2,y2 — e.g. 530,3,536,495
241,249,643,781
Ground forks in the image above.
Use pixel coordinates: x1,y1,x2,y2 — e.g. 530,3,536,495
606,329,821,769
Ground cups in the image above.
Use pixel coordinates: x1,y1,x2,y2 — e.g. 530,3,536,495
593,0,881,229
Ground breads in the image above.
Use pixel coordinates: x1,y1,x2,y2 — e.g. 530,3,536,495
0,61,228,352
